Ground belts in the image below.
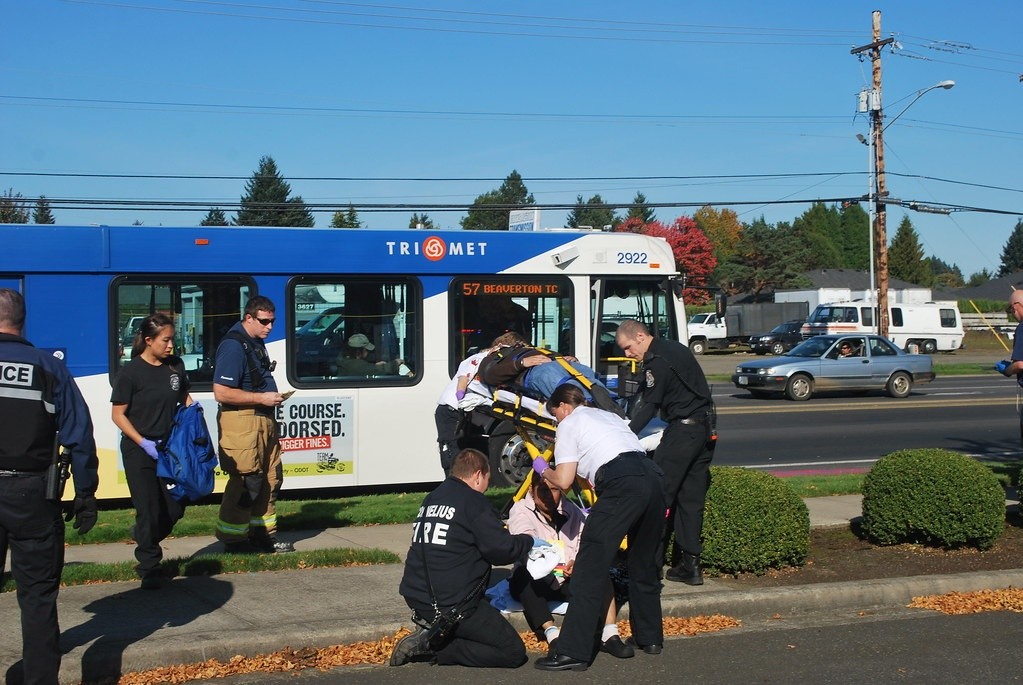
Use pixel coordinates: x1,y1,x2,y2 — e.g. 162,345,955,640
0,469,44,479
441,404,460,413
222,404,274,414
669,417,704,425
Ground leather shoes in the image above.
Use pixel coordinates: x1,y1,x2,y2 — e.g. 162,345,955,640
598,635,634,657
625,636,660,654
534,653,588,671
547,638,558,656
390,618,433,665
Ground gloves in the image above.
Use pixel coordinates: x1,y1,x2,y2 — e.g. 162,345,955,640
141,438,162,459
190,402,204,414
533,457,550,475
531,535,552,547
64,490,98,535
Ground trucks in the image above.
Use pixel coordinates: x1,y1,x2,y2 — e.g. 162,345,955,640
686,300,810,355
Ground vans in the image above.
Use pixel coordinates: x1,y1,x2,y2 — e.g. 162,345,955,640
799,299,966,355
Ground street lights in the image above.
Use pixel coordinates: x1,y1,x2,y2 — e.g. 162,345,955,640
864,79,958,338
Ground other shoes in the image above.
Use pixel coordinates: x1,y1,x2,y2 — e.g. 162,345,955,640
142,567,165,589
225,540,265,554
248,525,293,552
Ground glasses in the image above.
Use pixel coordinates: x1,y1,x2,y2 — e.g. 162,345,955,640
1005,302,1018,313
840,347,848,350
255,316,276,325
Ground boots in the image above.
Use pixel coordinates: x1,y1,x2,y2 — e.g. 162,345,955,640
665,549,703,584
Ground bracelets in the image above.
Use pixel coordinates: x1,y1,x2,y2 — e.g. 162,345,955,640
541,468,547,476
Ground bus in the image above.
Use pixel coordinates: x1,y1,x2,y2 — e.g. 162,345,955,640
0,219,692,514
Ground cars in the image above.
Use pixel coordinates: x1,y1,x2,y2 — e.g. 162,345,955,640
746,318,810,356
562,317,631,357
730,332,936,402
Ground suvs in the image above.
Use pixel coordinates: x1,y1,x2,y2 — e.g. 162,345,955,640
121,315,148,347
291,305,348,378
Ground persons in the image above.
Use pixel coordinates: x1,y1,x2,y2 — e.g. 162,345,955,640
110,313,193,590
615,319,716,585
213,295,293,554
838,342,852,357
334,333,406,375
532,383,666,671
0,288,100,685
390,448,535,667
478,343,643,420
507,465,634,657
435,331,527,475
995,289,1023,387
